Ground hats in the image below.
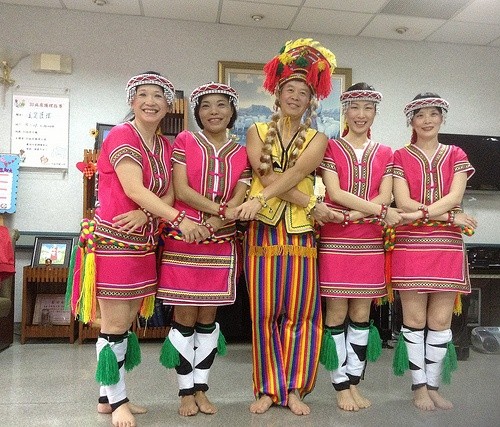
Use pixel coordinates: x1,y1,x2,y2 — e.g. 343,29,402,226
258,38,337,179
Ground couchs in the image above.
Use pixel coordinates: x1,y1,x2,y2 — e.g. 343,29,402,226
0,229,20,351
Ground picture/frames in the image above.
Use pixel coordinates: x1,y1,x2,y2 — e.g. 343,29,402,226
30,236,74,269
218,60,353,179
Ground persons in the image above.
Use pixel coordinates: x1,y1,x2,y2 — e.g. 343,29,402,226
393,92,478,409
63,71,204,427
156,83,251,416
246,38,336,415
319,82,404,411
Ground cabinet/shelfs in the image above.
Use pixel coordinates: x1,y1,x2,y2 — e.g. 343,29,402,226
21,264,79,344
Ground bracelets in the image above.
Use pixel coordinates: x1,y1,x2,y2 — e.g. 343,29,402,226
446,211,454,225
218,201,228,221
418,205,429,224
138,207,153,225
334,209,350,227
171,211,185,228
251,193,273,212
199,222,215,234
376,203,387,219
304,196,321,219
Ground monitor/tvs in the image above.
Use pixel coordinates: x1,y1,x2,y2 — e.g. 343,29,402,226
438,133,500,194
